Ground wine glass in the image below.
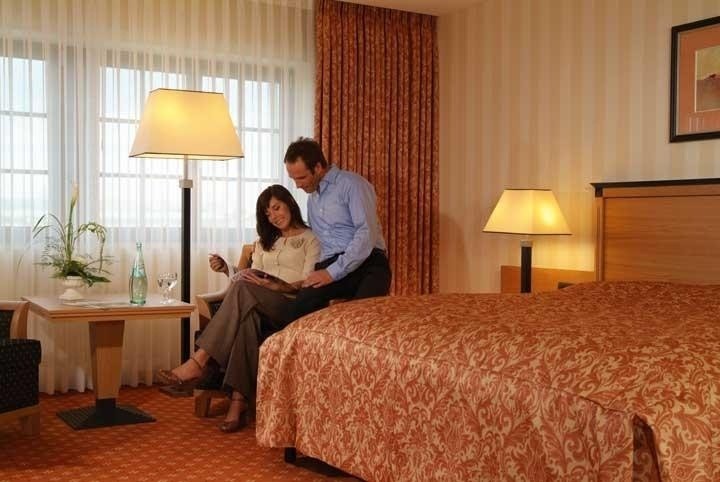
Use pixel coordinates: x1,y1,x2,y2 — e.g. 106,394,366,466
156,273,178,304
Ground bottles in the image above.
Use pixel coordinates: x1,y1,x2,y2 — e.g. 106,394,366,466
128,242,147,306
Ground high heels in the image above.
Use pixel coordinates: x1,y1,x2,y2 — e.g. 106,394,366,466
220,398,249,432
159,357,207,392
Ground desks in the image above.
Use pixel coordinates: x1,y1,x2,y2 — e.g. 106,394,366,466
22,293,198,431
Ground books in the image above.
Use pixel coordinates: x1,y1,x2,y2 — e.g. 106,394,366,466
208,253,297,293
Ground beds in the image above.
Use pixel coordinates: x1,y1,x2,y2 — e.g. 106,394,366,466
255,178,720,482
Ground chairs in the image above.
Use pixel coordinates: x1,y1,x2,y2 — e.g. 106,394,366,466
194,245,255,417
0,301,42,437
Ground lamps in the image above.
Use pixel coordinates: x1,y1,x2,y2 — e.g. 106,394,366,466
130,88,245,399
483,189,573,293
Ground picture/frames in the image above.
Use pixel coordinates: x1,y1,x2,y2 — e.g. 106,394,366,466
669,16,720,143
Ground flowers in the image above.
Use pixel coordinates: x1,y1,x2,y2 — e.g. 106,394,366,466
16,182,116,286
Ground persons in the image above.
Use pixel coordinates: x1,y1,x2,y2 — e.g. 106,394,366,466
282,137,392,318
154,184,322,433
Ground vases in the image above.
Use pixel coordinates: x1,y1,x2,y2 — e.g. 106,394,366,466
59,276,84,300
